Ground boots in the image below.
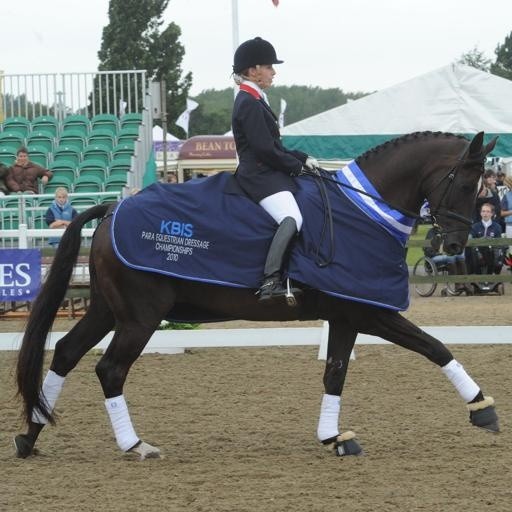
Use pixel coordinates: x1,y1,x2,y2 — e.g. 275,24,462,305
257,217,304,304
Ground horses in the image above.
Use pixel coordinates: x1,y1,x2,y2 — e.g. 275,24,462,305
14,128,501,464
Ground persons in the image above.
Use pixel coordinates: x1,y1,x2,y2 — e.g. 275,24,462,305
469,202,506,275
230,36,320,304
478,159,512,270
43,187,79,245
0,161,10,197
424,224,474,293
7,147,54,195
166,171,178,184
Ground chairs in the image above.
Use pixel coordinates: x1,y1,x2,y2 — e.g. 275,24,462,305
0,111,148,240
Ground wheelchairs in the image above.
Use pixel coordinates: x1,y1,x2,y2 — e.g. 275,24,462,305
470,245,508,296
412,247,469,298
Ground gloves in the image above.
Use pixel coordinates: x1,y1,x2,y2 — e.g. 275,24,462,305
306,156,319,170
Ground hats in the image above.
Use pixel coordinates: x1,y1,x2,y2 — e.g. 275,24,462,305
232,37,283,72
